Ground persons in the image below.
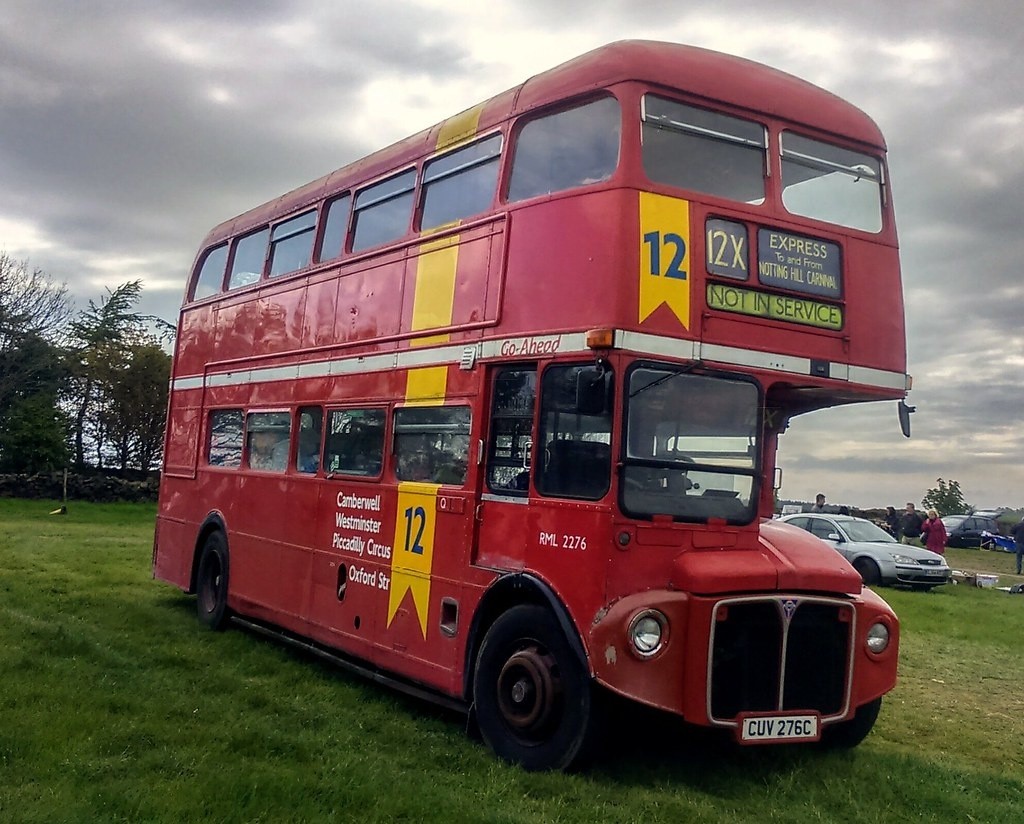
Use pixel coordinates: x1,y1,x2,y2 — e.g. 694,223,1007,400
900,502,924,545
881,505,899,542
1010,516,1024,575
836,506,850,534
811,494,825,514
921,510,947,558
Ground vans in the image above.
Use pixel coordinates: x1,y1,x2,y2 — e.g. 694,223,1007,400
920,510,1004,548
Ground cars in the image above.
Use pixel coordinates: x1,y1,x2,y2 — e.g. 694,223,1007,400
774,514,951,591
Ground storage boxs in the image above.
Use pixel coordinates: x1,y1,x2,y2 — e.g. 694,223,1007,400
950,568,999,590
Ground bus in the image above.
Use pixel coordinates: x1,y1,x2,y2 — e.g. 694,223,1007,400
152,38,916,773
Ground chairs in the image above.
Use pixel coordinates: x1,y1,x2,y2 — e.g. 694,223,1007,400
547,439,612,499
330,433,356,470
272,439,301,473
400,452,433,482
